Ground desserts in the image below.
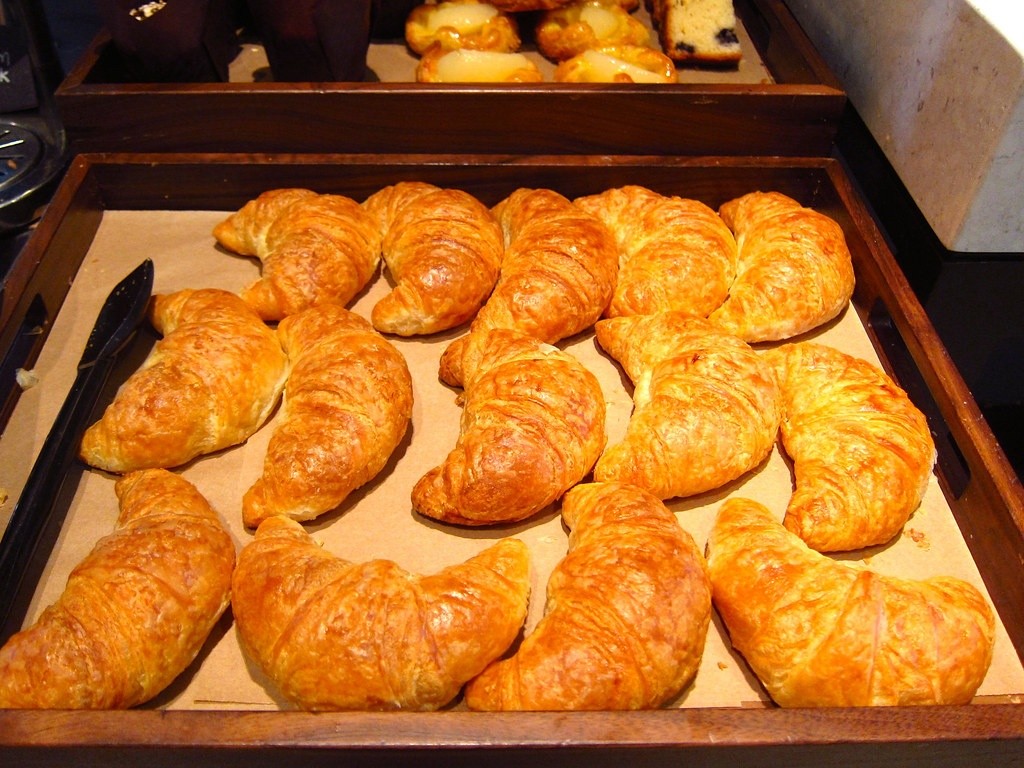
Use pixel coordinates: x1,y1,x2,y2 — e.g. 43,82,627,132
404,0,675,87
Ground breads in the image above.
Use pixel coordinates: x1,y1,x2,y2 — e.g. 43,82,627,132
0,189,996,710
651,0,742,66
